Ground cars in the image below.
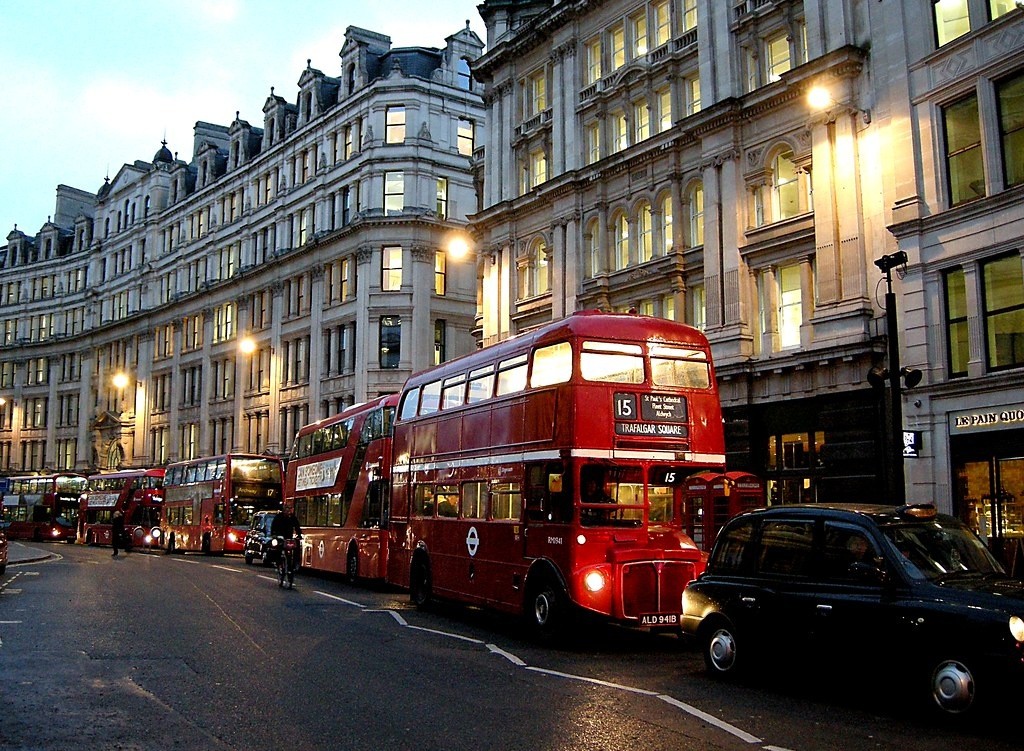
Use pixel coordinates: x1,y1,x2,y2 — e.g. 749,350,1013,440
679,501,1024,728
243,510,283,566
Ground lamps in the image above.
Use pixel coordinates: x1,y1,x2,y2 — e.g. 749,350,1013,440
808,84,871,125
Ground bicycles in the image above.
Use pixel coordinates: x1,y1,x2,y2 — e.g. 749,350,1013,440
274,535,302,590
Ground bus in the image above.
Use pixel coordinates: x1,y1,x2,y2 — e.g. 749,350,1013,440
281,395,399,589
383,310,761,643
77,468,165,549
0,472,88,544
158,452,284,556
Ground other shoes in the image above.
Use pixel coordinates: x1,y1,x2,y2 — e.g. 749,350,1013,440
111,551,118,556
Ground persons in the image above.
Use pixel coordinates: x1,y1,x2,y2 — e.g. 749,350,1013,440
111,511,131,556
833,532,883,585
271,505,300,578
